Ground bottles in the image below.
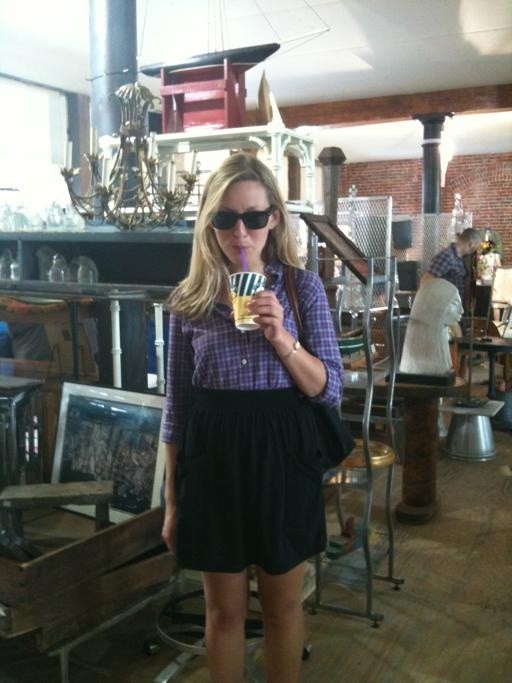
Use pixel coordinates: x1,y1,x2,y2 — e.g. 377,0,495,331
76,257,99,283
48,253,71,282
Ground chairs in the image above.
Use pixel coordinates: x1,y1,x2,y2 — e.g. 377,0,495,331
321,252,405,627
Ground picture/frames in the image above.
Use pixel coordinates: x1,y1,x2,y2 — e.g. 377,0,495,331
52,380,168,523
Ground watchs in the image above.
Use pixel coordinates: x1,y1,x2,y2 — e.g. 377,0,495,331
282,340,301,361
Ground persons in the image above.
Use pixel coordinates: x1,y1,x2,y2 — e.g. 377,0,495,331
161,154,344,683
418,228,482,378
399,278,464,375
473,241,501,320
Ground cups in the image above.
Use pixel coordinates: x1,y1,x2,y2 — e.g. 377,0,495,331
227,272,267,331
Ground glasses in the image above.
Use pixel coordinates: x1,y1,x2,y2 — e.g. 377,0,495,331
212,205,276,230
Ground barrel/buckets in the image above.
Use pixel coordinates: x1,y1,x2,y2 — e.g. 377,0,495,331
445,410,500,464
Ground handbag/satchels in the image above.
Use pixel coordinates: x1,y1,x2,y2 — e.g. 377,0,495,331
295,398,355,469
